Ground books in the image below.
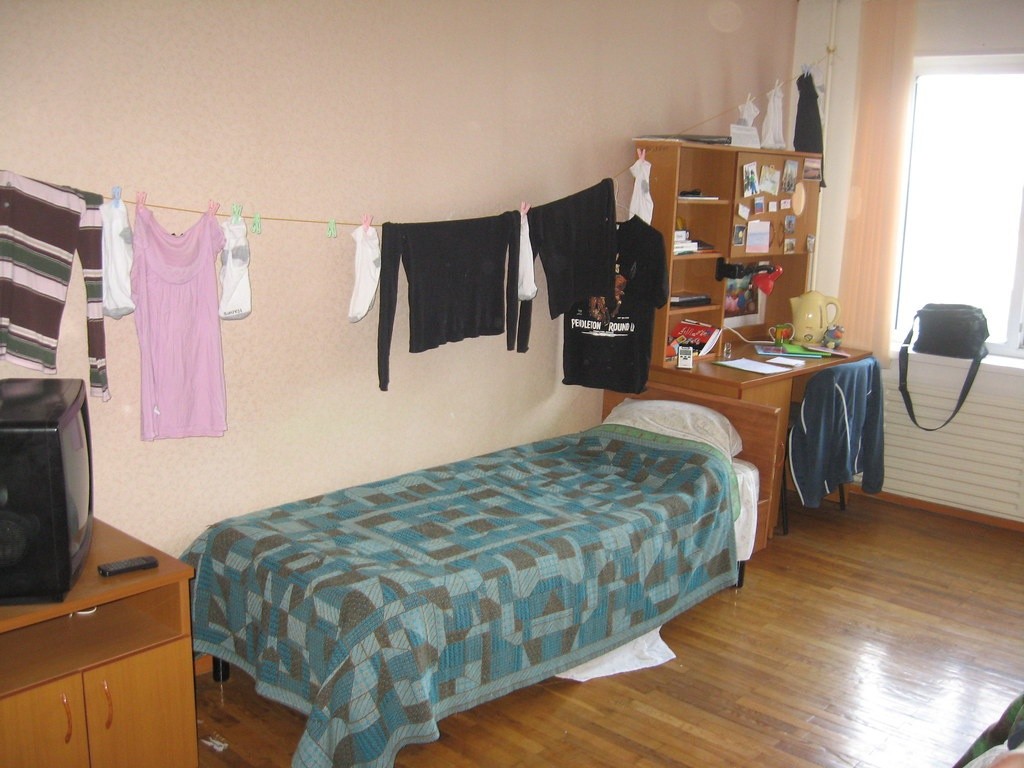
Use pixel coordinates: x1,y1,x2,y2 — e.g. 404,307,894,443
672,319,722,357
755,344,852,358
678,196,719,200
692,239,719,253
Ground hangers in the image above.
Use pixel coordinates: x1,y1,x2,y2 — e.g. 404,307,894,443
608,175,632,218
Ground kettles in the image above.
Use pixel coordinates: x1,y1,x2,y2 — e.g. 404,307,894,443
789,290,840,344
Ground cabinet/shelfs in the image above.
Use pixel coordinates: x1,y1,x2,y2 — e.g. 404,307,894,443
628,137,823,364
0,518,201,768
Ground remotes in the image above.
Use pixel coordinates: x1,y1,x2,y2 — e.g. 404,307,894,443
97,556,159,577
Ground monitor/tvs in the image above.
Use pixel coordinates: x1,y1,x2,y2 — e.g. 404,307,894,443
0,378,96,605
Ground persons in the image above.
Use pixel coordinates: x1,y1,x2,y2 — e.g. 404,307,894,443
750,170,758,194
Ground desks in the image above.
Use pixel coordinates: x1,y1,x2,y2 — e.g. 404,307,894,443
600,349,873,541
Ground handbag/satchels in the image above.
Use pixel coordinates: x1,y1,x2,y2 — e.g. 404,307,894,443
899,303,989,430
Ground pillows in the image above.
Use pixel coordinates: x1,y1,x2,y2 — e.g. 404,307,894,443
604,398,742,459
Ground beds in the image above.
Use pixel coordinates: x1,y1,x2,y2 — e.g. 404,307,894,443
175,427,760,768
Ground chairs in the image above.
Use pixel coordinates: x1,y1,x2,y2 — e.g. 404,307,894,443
778,357,885,536
